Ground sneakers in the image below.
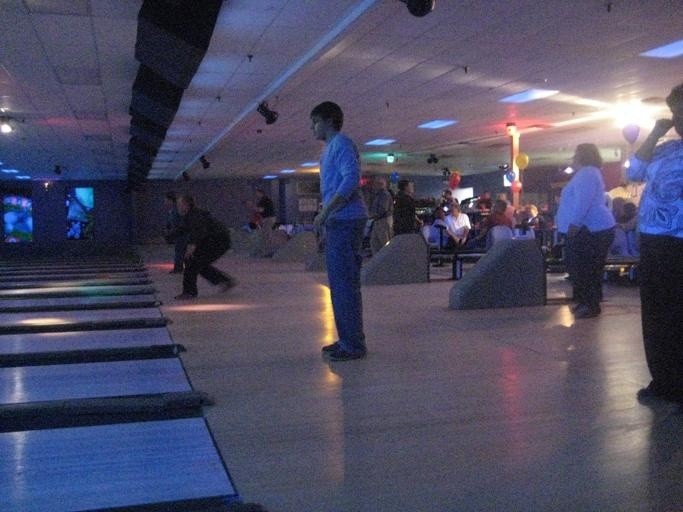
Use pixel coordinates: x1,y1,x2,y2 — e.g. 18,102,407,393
219,279,240,293
322,341,340,352
330,347,366,361
173,292,195,300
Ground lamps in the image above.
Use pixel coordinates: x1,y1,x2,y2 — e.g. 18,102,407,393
182,171,191,183
387,153,395,163
507,122,516,136
257,104,278,124
199,156,211,170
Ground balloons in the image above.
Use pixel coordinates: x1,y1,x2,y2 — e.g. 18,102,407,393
622,123,640,144
389,171,399,185
448,172,461,189
505,170,516,182
360,175,368,186
511,180,522,193
515,152,529,170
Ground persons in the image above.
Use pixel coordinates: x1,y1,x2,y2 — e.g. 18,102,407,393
428,207,449,248
367,174,393,256
625,84,683,403
519,204,545,242
393,180,424,234
612,196,625,220
617,203,639,231
241,199,260,232
554,143,616,318
444,202,472,249
163,190,184,274
477,190,493,209
253,189,278,239
308,100,369,362
174,193,233,300
491,191,511,210
442,189,459,215
466,199,513,248
